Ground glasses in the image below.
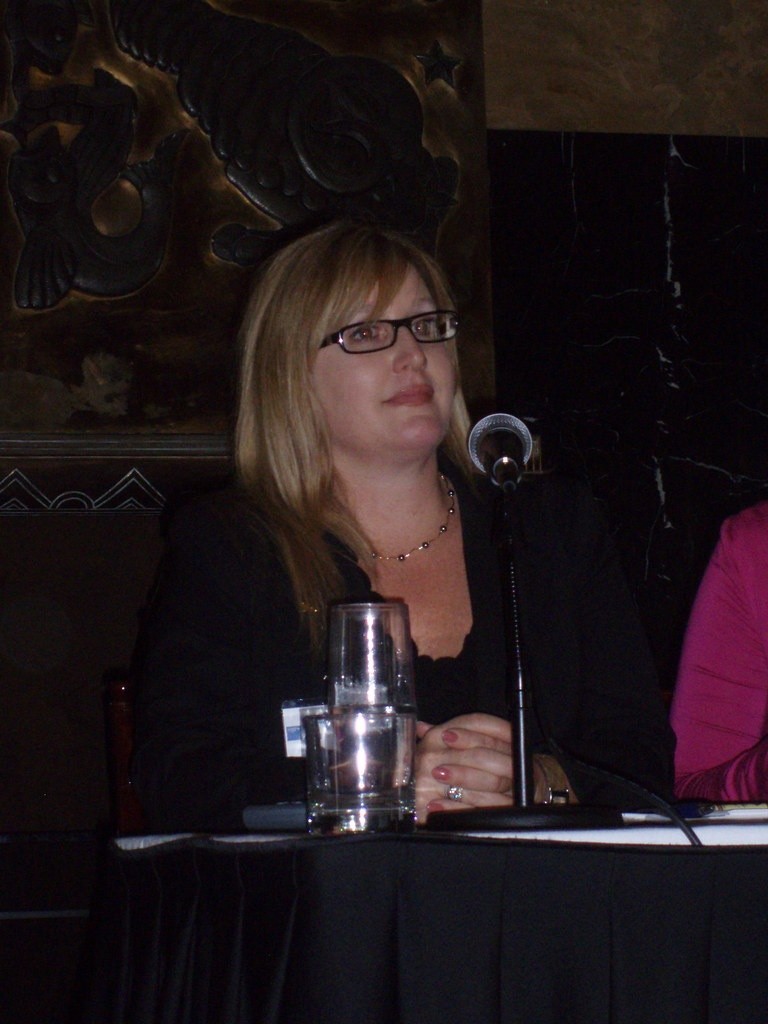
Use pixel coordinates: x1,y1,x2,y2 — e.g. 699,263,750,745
318,309,466,355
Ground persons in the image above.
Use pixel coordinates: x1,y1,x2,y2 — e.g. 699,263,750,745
670,499,768,800
134,228,675,836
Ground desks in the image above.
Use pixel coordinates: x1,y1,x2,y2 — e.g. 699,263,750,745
69,818,768,1024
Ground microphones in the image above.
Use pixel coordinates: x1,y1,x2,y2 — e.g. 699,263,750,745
468,413,532,492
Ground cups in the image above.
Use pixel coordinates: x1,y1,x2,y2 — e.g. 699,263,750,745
329,601,415,709
300,712,416,837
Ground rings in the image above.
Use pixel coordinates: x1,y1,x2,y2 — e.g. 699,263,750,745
448,786,462,799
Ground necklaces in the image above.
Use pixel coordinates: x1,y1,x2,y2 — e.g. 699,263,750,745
371,472,455,560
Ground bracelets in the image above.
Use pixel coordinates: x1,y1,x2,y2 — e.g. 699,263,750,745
534,754,570,806
